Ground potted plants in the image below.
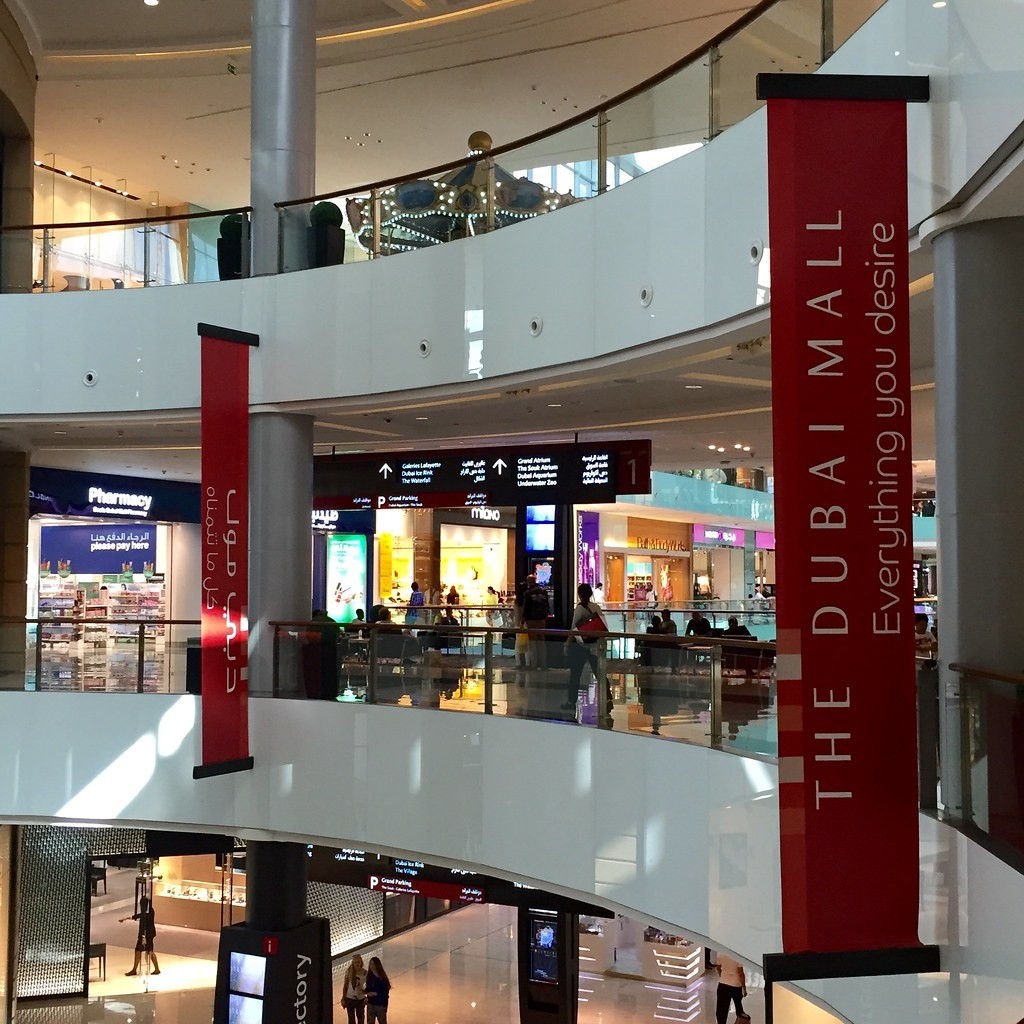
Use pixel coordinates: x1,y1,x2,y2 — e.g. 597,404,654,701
307,201,346,270
217,213,250,280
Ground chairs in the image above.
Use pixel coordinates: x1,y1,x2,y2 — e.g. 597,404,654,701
91,868,107,896
90,942,106,981
333,631,467,690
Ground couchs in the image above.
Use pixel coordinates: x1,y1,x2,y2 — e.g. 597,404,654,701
635,628,778,675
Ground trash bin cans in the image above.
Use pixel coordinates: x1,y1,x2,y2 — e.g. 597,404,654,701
304,201,346,269
185,636,202,696
215,214,251,282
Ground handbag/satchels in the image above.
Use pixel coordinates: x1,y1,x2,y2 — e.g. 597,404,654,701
575,602,608,644
515,625,529,655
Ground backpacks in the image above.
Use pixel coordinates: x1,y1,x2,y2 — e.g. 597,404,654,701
526,587,549,621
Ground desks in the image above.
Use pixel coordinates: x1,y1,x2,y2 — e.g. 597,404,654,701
349,637,371,686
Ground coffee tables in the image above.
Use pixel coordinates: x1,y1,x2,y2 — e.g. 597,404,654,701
689,644,712,675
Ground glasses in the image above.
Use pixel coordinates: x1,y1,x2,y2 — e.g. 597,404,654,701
352,954,360,957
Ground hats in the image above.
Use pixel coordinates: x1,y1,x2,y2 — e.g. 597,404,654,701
527,574,537,583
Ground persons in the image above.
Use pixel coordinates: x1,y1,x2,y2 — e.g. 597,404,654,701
593,583,605,603
341,954,368,1024
560,583,614,715
364,957,392,1024
487,587,499,605
118,896,160,976
643,582,775,676
511,574,549,671
312,586,459,657
715,951,751,1024
405,582,424,623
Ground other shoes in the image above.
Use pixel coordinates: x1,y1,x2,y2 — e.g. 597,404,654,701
125,970,136,976
560,702,576,710
511,665,522,670
151,970,160,974
606,700,614,714
